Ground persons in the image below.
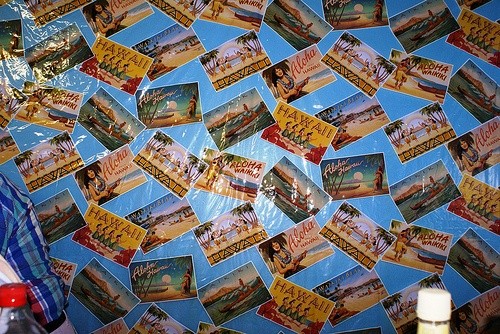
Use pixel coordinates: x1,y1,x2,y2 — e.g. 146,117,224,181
0,1,497,328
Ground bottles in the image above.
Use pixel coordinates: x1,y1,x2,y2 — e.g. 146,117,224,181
0,281,50,334
